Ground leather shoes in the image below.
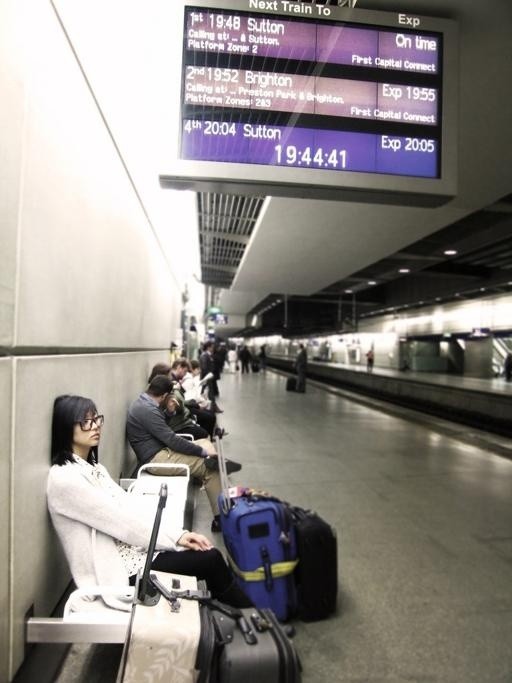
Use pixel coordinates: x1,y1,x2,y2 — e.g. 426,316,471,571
211,520,221,532
205,454,241,472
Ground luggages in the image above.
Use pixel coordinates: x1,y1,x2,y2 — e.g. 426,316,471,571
286,377,296,391
212,607,303,683
215,428,296,622
285,506,337,623
116,482,219,683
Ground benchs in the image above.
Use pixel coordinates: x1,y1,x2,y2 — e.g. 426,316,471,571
26,433,194,645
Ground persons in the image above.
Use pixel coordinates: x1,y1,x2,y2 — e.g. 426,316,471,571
46,394,295,638
366,350,373,372
311,338,362,364
291,343,308,393
506,354,512,380
125,341,267,532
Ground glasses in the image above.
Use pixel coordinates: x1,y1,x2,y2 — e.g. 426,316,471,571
78,415,104,431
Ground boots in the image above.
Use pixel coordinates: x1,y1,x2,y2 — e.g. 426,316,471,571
210,402,223,413
